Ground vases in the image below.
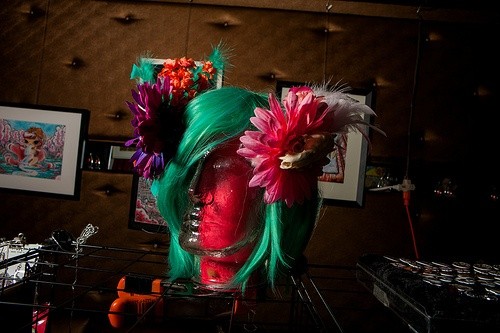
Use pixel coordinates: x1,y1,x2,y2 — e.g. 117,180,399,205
278,200,319,277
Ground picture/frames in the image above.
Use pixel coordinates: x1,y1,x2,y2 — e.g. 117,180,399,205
277,80,374,210
129,168,171,234
0,101,90,200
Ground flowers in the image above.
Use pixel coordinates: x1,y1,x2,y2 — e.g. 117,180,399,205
237,86,372,208
126,56,217,176
